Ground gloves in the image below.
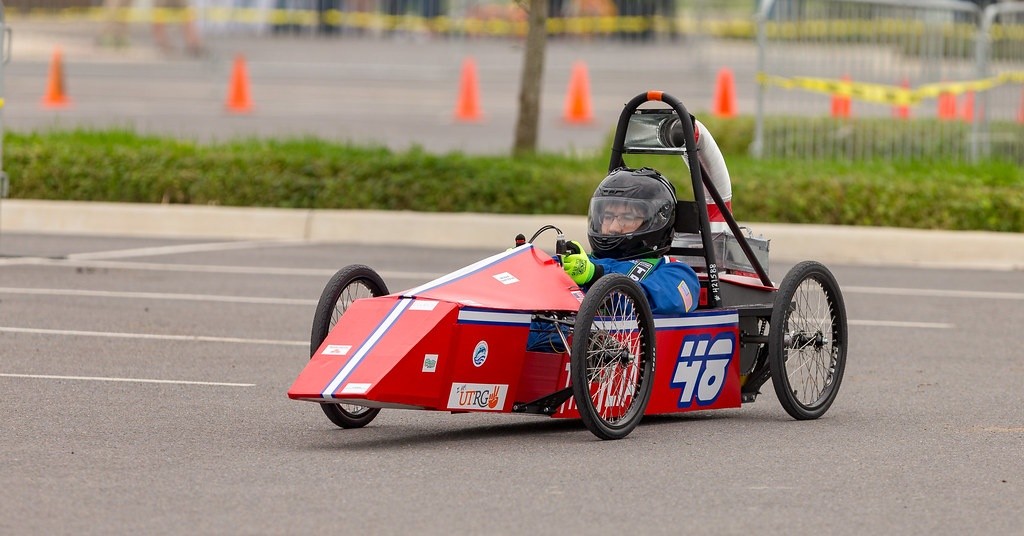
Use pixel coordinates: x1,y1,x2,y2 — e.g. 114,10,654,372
563,240,598,287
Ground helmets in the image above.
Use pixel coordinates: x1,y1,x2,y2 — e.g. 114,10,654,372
587,166,676,261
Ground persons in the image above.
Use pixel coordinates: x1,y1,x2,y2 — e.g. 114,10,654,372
561,169,701,318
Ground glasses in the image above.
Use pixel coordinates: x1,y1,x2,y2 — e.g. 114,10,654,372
598,212,646,226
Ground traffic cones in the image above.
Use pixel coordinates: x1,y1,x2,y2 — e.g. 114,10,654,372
451,59,484,125
892,80,913,121
563,63,596,125
828,75,852,120
225,57,254,112
1016,87,1024,121
959,87,984,124
937,83,959,122
41,50,75,110
713,68,736,118
